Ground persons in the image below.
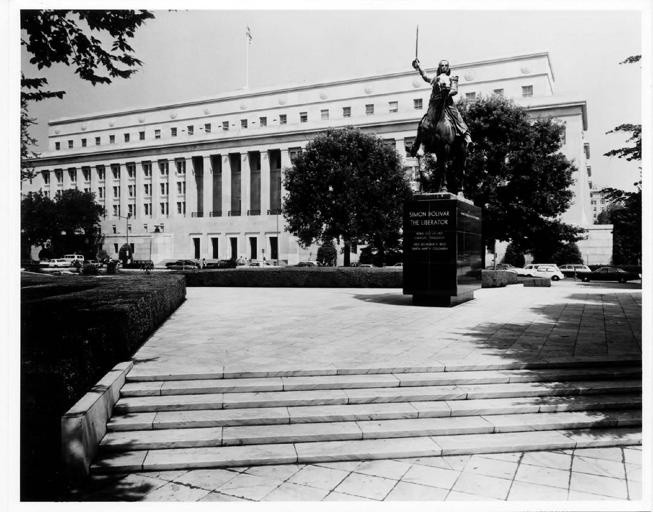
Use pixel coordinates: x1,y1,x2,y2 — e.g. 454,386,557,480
402,57,477,156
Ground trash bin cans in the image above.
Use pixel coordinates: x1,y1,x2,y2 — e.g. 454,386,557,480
107,260,120,274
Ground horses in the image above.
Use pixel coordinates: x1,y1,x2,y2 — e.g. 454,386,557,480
417,73,466,197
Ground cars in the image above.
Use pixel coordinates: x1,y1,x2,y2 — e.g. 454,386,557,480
486,261,641,284
47,253,103,268
